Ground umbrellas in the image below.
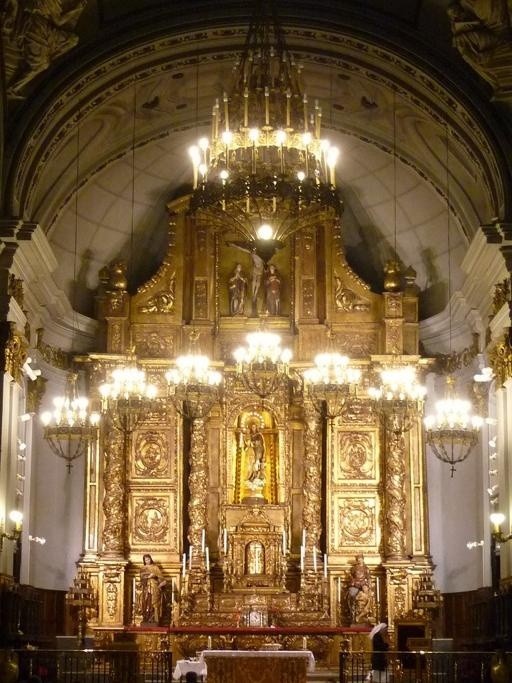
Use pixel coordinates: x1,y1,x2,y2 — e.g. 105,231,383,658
368,621,389,643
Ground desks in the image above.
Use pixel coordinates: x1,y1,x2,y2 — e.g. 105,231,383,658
175,659,206,682
203,651,313,682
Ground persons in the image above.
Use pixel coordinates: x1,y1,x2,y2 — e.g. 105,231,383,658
244,422,268,481
263,262,284,317
448,0,512,95
138,551,169,622
225,261,248,317
0,0,88,106
226,238,274,302
369,631,390,683
345,552,375,616
352,581,375,623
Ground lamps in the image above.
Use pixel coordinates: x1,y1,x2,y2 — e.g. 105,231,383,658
162,351,225,424
489,512,512,543
229,330,293,405
37,393,102,478
0,510,26,544
299,353,362,426
360,367,426,451
97,366,159,445
186,0,337,265
422,393,489,478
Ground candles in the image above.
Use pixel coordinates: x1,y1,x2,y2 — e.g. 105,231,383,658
208,636,211,647
282,529,327,580
180,527,229,576
338,576,341,604
172,578,174,603
133,577,136,603
376,576,380,602
303,637,306,648
